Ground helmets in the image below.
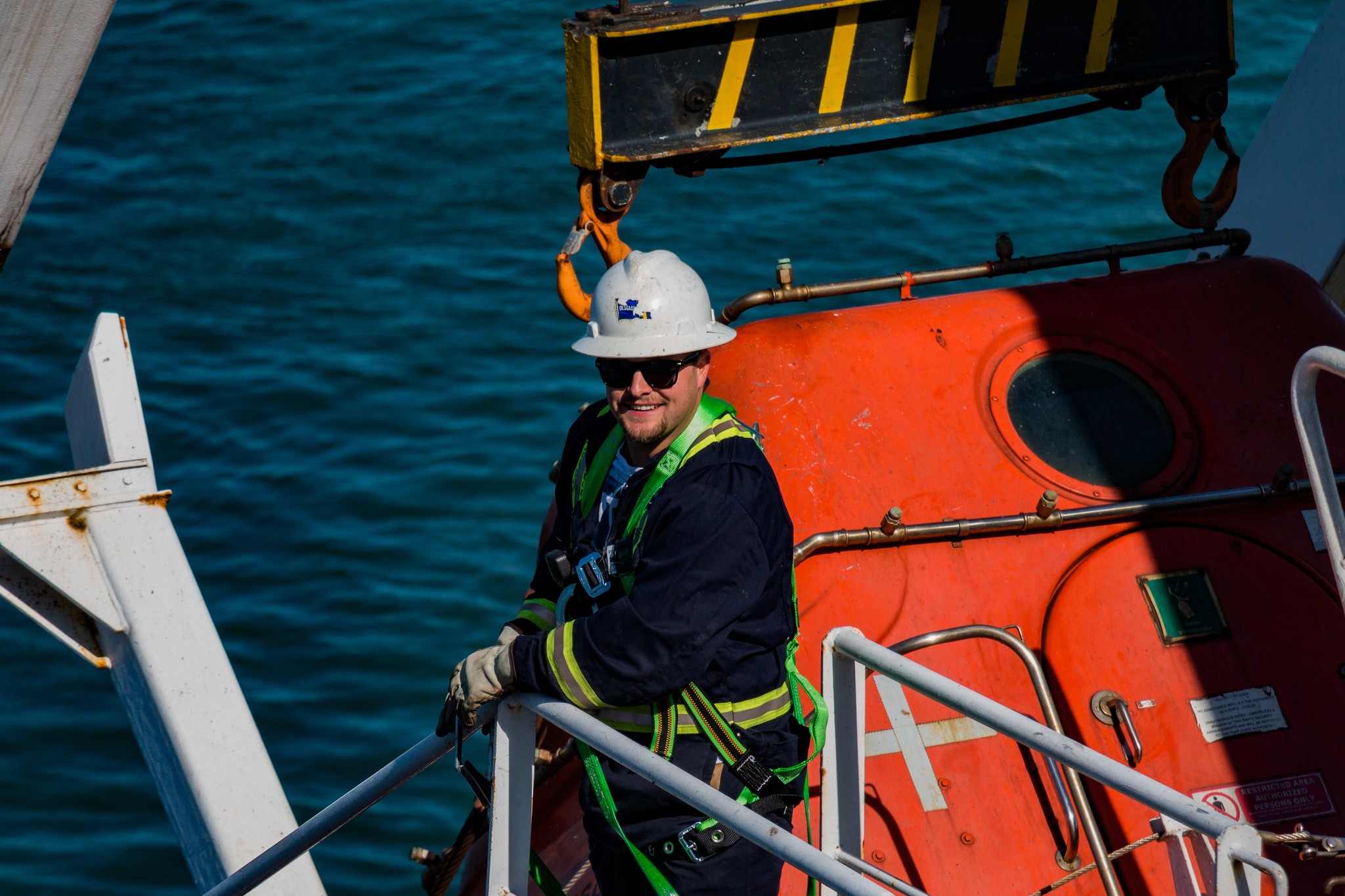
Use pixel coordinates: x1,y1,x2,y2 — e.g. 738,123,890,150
571,249,736,357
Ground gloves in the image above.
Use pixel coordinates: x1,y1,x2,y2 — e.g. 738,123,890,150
482,622,524,736
436,640,519,738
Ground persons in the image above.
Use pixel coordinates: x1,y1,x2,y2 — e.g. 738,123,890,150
434,246,814,896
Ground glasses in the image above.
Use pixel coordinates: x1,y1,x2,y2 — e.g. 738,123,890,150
595,349,706,388
